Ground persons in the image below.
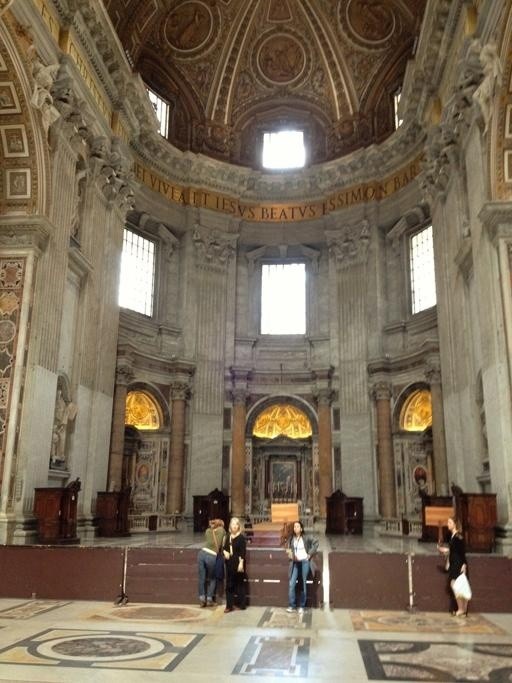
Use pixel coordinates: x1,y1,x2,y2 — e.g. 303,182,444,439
445,517,469,618
470,38,503,138
197,519,227,607
286,522,319,614
34,63,61,151
222,517,246,613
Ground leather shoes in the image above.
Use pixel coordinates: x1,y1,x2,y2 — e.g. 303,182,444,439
224,607,230,613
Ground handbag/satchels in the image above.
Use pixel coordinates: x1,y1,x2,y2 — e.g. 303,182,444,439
214,553,224,581
453,573,472,601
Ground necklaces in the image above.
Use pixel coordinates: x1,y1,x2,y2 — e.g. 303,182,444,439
295,536,302,552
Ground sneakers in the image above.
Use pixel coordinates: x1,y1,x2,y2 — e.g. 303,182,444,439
200,600,218,607
286,607,304,614
